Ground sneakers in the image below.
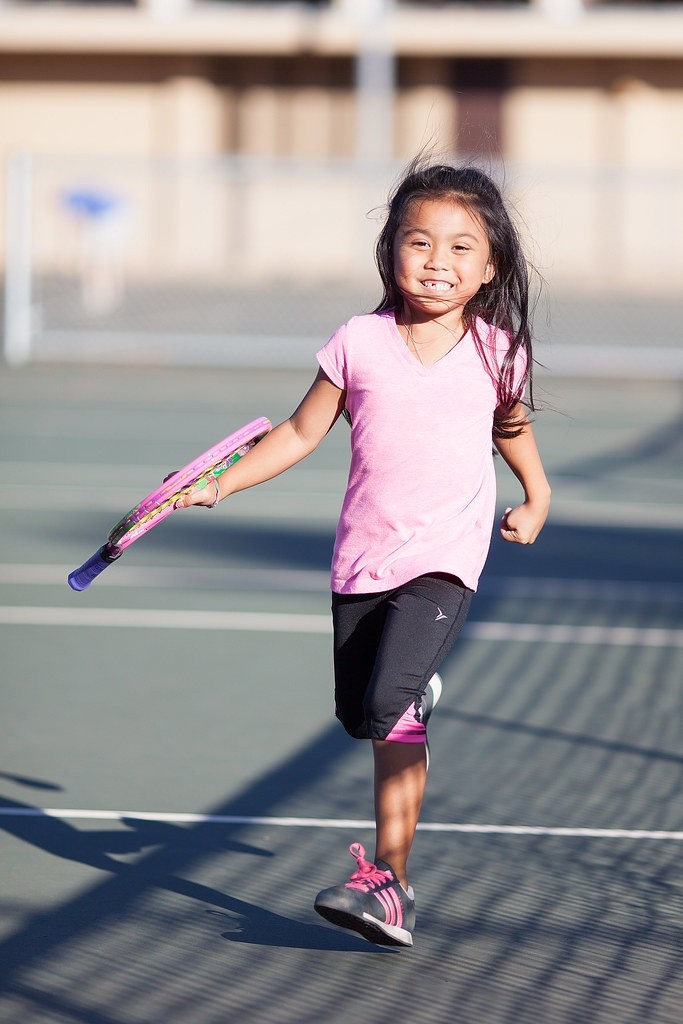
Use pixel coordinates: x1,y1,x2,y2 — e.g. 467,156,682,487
313,858,418,947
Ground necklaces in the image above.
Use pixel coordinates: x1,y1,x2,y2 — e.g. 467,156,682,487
401,311,467,366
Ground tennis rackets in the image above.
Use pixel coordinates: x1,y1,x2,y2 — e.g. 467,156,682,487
67,416,271,591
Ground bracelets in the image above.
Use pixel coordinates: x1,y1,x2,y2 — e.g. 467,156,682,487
206,478,220,509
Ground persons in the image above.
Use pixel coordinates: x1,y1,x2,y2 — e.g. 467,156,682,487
162,140,550,946
